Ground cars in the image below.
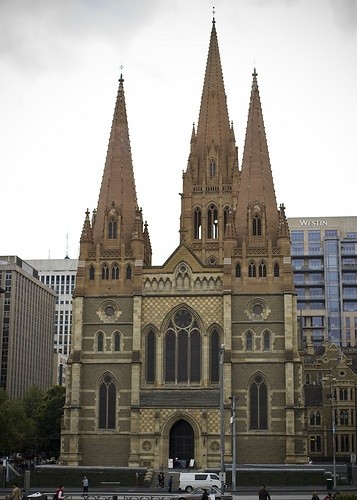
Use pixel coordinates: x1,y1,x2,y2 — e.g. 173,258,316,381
190,486,222,496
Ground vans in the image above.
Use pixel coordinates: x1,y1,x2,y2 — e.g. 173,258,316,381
177,472,227,493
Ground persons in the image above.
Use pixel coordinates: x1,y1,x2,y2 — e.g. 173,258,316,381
155,472,165,488
312,492,319,500
82,476,88,497
6,483,27,500
324,492,334,500
202,489,208,500
168,476,173,493
56,485,66,500
259,485,270,500
113,496,117,500
